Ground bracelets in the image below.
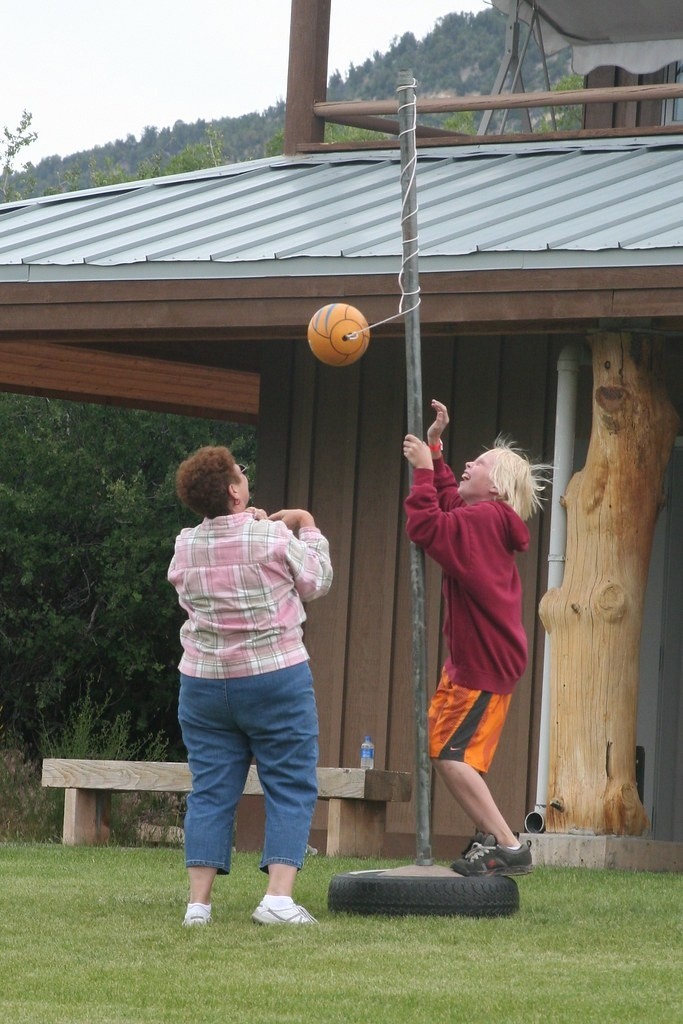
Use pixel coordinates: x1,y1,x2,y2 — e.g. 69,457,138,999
427,439,444,452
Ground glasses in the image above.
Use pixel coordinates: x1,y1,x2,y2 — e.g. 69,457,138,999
239,464,248,474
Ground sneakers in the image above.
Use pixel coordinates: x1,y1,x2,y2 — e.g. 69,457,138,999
181,903,212,927
251,904,320,928
450,831,533,877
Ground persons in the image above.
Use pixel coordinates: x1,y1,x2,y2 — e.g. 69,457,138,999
404,399,549,879
168,445,334,929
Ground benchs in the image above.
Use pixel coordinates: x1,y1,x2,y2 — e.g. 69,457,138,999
42,757,413,857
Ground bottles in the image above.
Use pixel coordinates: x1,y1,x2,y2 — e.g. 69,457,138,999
361,736,374,769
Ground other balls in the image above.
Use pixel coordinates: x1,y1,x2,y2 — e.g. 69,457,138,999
308,304,369,365
